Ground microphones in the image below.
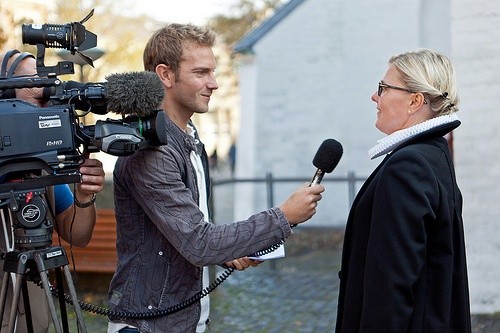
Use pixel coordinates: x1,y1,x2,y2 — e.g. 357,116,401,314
69,70,166,114
293,139,343,227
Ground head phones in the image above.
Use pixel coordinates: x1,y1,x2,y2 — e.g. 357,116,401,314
0,49,36,98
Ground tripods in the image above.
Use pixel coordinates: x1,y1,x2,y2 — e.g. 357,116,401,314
0,187,86,333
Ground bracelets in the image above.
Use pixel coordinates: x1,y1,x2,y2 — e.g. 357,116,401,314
74,191,96,209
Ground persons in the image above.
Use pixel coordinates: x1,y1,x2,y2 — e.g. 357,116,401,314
333,50,472,333
107,22,325,333
0,50,105,333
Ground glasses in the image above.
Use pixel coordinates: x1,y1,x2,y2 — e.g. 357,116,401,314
378,79,427,104
